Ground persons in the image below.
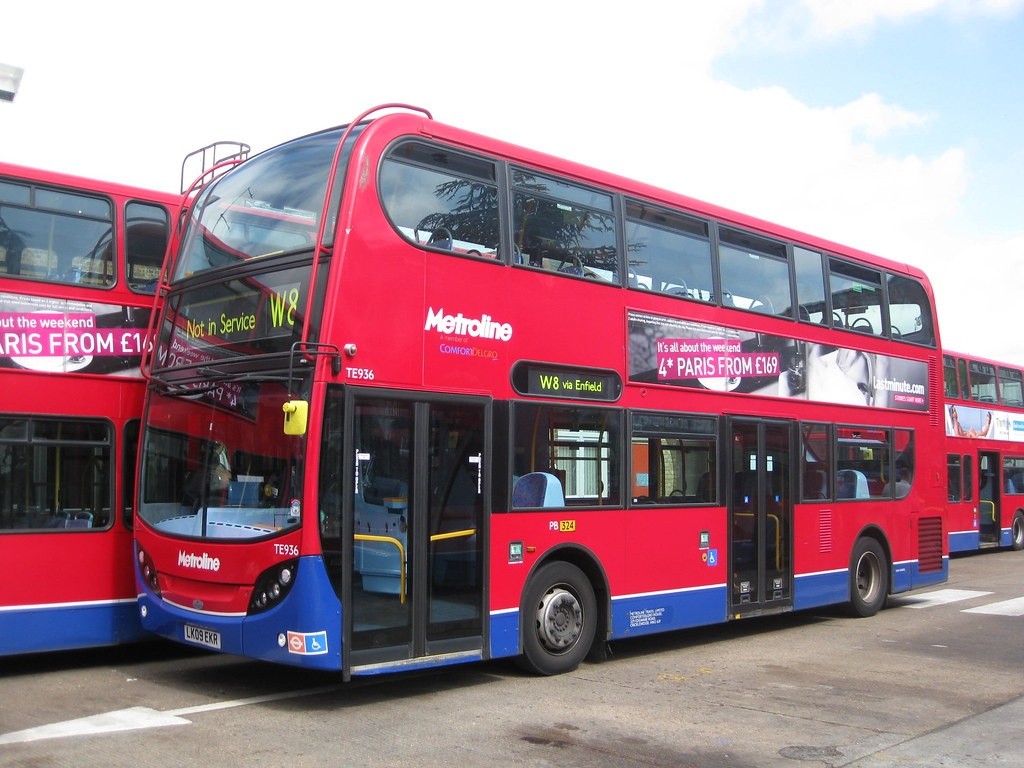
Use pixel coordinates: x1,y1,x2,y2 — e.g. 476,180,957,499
882,467,909,497
950,405,991,437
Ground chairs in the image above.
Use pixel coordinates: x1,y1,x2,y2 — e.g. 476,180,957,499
139,278,164,295
1005,478,1017,493
944,388,1024,406
425,226,903,343
42,509,93,529
697,468,912,499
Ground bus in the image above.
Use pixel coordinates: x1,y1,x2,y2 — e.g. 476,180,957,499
131,102,948,673
0,163,554,655
731,347,1024,555
